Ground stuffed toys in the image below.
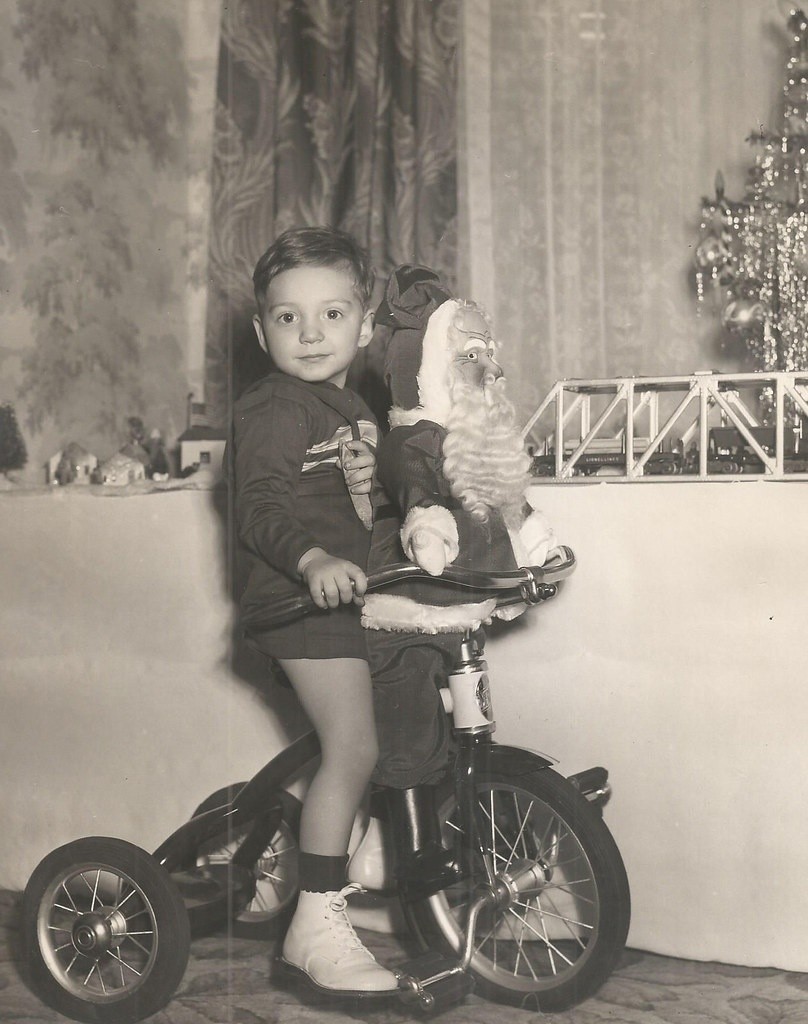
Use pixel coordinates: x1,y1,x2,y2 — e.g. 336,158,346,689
356,265,566,902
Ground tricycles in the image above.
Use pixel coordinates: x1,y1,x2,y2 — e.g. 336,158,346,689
14,544,634,1024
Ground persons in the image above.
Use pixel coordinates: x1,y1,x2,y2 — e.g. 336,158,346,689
224,225,403,998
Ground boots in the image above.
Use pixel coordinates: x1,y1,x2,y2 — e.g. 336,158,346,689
348,815,397,893
269,882,400,1008
